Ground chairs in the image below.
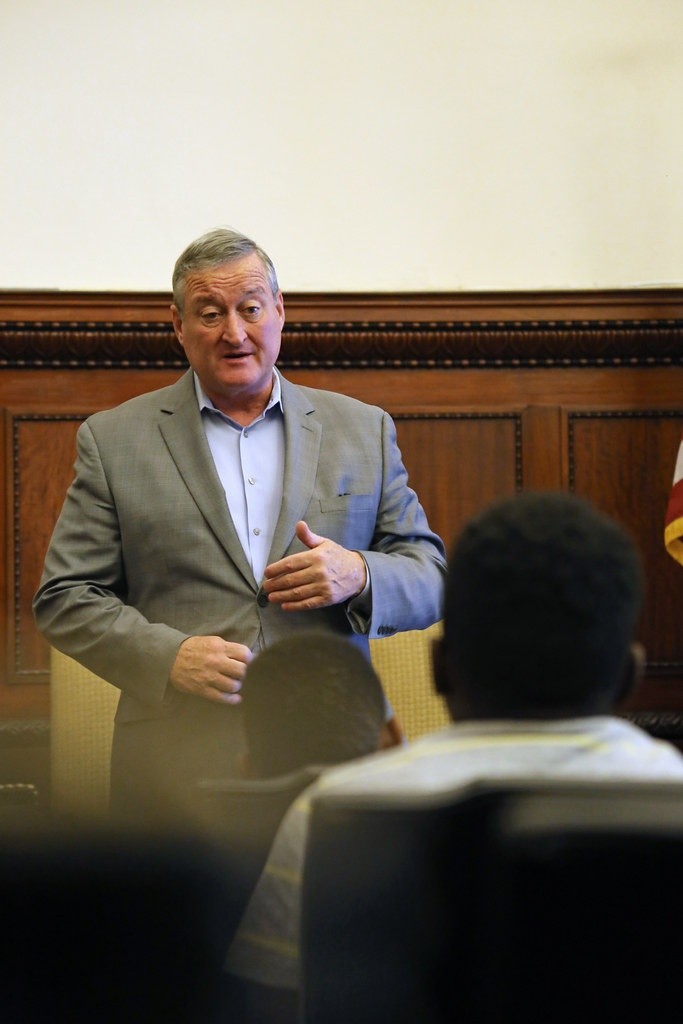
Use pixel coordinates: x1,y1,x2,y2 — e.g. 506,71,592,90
296,780,683,1023
195,770,319,905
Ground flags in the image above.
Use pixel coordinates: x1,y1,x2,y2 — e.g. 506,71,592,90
664,436,683,566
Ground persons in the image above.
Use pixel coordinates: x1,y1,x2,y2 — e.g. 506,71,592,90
32,228,447,843
224,492,683,1023
